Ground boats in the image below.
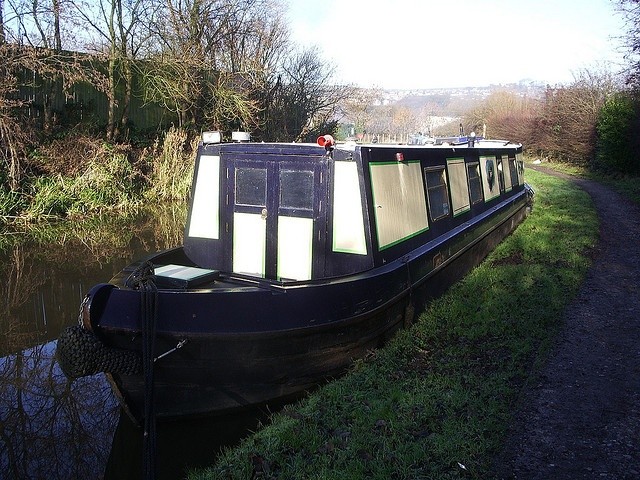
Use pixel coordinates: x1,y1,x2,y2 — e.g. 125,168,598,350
59,131,534,421
